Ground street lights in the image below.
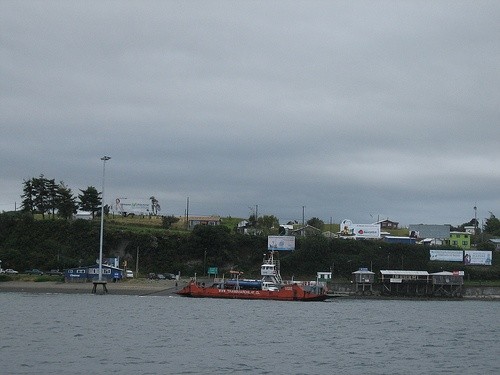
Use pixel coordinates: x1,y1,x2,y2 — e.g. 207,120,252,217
473,206,477,228
98,156,112,280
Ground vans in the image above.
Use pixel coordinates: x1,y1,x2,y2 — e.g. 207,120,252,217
126,269,134,279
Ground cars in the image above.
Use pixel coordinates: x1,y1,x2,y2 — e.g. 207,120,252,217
46,270,63,277
148,272,176,280
1,268,19,276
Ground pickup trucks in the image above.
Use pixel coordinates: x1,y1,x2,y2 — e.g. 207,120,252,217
24,268,44,276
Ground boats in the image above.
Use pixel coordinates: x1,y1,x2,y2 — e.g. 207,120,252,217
172,240,330,302
